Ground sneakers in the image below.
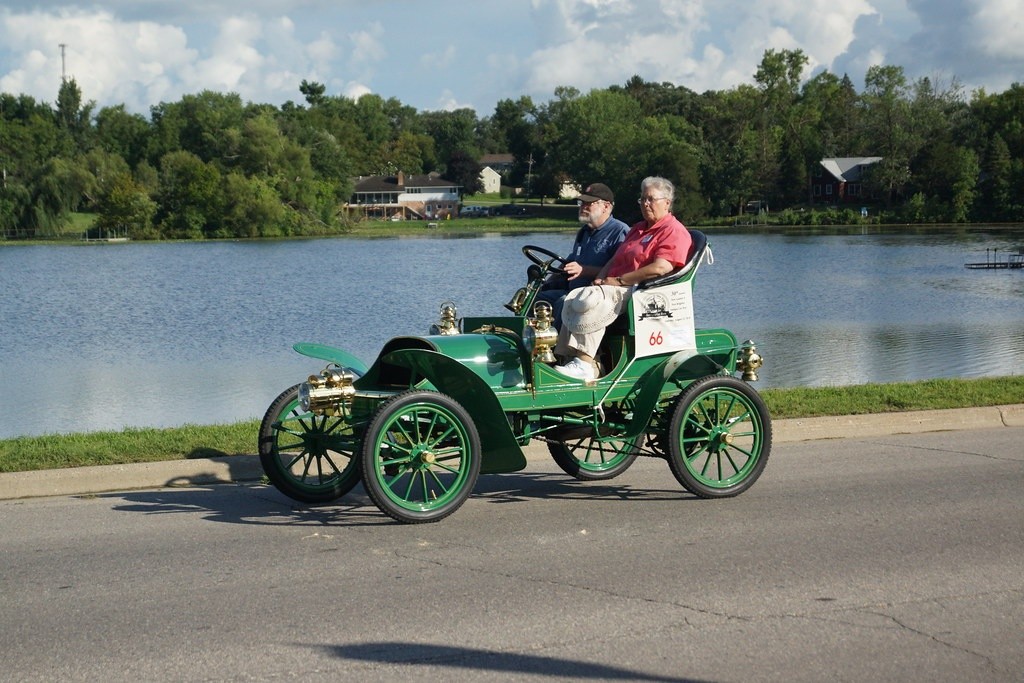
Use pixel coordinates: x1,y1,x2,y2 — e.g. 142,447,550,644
554,357,595,381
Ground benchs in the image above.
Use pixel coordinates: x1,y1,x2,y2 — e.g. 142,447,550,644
605,228,709,336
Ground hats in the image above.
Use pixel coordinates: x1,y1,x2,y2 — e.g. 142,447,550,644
577,183,614,203
561,285,623,335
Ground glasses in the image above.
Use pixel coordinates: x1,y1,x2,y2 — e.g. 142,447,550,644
576,200,607,207
638,197,667,204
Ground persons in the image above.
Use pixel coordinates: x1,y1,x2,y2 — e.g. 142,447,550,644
553,176,692,381
515,183,631,335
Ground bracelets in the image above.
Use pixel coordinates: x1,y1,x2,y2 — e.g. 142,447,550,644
616,277,622,284
